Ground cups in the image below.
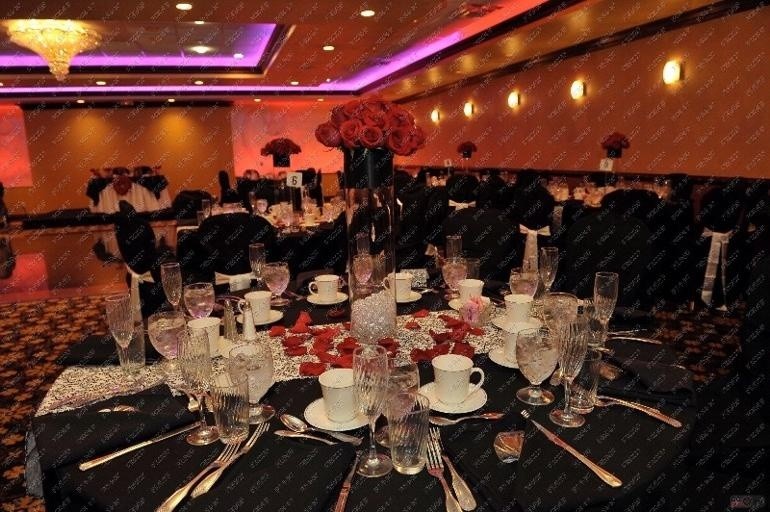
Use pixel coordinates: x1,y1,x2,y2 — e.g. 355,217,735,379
383,391,433,476
424,167,609,209
195,189,343,233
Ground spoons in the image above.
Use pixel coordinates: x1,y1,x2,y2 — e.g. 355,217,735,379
279,412,364,446
590,397,683,431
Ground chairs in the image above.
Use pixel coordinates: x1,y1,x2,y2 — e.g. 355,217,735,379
113,164,769,326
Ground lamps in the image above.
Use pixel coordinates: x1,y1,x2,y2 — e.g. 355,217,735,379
5,18,102,83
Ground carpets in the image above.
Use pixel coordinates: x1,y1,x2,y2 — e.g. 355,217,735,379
1,288,743,512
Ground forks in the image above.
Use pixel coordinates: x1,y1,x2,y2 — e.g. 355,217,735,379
420,425,477,511
156,424,270,511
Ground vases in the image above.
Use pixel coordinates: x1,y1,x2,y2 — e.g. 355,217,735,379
272,153,292,204
342,146,400,342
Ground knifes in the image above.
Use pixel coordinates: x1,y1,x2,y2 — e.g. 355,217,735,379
530,417,625,487
79,417,205,471
334,451,362,512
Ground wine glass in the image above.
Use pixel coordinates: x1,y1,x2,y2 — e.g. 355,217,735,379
103,242,295,446
350,232,559,294
351,345,421,478
516,272,620,428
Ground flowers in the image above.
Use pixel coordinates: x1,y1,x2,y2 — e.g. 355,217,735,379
601,132,631,160
457,141,478,158
261,138,303,167
318,95,427,188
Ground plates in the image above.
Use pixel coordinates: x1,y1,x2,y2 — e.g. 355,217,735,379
305,272,540,432
